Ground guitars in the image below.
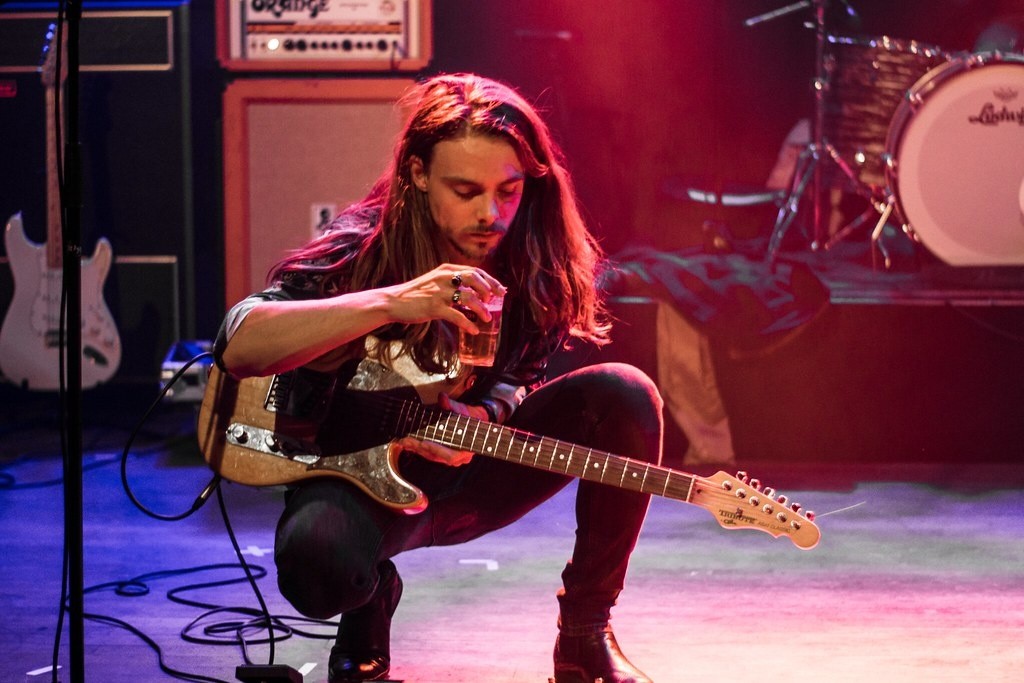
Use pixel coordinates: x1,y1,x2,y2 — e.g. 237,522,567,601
0,19,122,391
198,332,821,550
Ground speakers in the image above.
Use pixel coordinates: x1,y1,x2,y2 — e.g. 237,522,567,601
0,0,220,430
223,78,420,314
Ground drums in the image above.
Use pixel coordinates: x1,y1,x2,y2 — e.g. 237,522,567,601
800,35,951,197
885,51,1024,267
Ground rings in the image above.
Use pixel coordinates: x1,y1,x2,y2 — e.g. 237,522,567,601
452,290,461,302
451,273,462,289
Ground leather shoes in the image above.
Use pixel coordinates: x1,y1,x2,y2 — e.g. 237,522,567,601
553,631,653,683
328,561,403,683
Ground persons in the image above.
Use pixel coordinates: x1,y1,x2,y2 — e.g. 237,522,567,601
213,71,665,683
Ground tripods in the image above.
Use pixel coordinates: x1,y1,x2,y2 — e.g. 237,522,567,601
742,0,882,278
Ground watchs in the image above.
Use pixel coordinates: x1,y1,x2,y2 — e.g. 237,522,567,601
470,398,499,424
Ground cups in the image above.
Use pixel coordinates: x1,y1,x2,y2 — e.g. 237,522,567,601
458,287,507,367
